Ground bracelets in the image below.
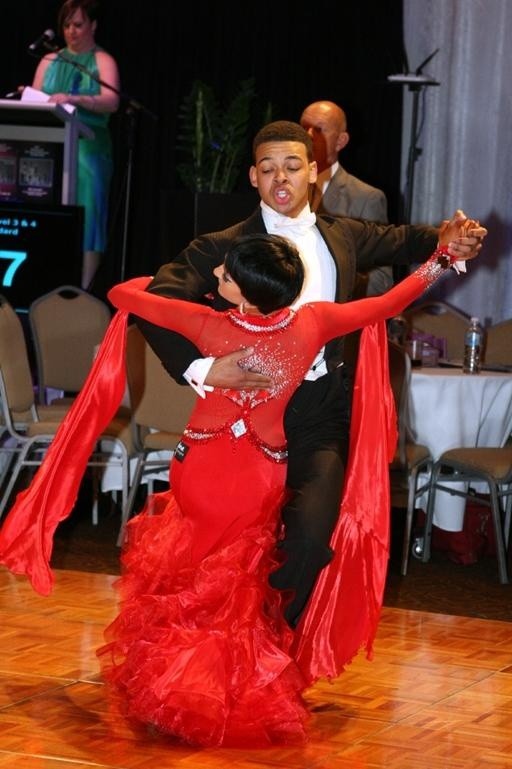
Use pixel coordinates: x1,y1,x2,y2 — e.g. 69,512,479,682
78,94,85,105
89,95,97,111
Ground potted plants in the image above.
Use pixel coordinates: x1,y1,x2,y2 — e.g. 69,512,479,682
174,79,273,258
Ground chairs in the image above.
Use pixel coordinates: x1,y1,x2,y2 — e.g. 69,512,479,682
0,286,512,584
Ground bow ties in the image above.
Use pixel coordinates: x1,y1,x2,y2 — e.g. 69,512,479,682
274,212,317,232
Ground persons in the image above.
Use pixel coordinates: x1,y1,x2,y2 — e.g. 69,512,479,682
130,121,490,630
300,99,393,297
105,207,487,751
16,0,121,290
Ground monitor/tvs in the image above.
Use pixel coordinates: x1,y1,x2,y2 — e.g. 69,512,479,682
0,202,86,314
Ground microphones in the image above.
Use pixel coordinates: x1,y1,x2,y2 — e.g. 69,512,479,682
29,29,55,49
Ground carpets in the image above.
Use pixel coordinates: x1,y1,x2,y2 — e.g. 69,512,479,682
50,516,512,622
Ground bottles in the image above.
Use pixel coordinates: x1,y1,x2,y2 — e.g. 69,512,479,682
462,317,484,375
404,340,422,367
422,342,439,368
